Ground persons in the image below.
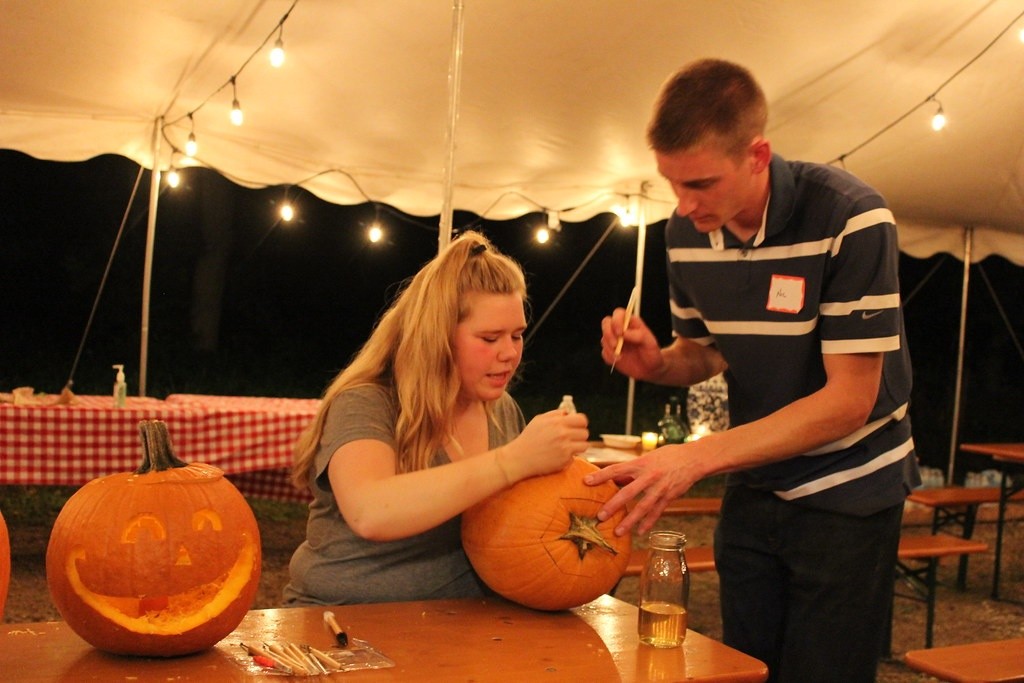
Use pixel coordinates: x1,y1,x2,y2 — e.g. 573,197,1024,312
282,229,589,609
581,57,923,682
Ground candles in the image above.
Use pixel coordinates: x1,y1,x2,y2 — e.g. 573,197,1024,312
641,430,658,451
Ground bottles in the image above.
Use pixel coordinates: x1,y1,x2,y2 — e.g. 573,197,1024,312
557,395,577,415
637,530,690,649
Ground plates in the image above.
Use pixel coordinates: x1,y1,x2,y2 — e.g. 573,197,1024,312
599,433,641,449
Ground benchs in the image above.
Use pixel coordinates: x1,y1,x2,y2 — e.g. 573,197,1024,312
609,547,717,575
905,488,1024,594
904,637,1024,683
890,533,989,647
629,498,722,514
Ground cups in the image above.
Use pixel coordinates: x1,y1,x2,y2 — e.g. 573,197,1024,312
642,432,658,451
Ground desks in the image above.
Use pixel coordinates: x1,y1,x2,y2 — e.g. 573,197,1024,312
584,440,638,466
960,443,1024,601
0,393,322,504
0,594,767,683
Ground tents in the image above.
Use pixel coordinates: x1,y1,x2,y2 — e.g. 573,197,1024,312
1,0,1023,485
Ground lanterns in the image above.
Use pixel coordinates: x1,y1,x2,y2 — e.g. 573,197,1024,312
45,418,262,657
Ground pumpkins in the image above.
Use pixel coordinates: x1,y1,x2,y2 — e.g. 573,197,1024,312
0,511,12,620
459,455,630,612
